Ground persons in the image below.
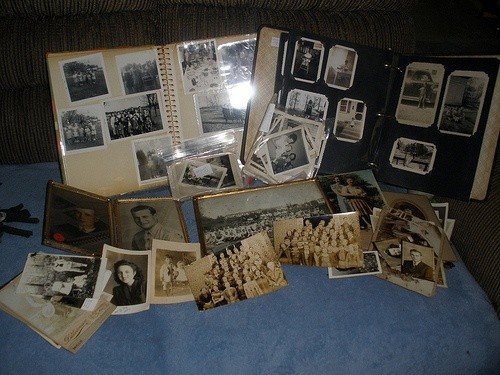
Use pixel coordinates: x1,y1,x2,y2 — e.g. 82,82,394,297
223,99,248,126
417,82,429,109
160,255,177,297
400,204,415,216
204,200,327,250
340,60,350,70
197,244,287,310
382,215,429,247
49,195,111,253
181,43,217,87
276,218,363,268
268,133,296,175
446,103,478,134
402,249,434,282
63,120,98,147
130,205,183,251
330,176,366,229
43,257,90,297
110,105,152,140
304,100,315,119
394,142,417,168
173,260,188,285
302,48,312,69
136,147,168,181
121,60,161,95
110,260,147,306
73,70,96,87
350,105,356,128
335,253,379,272
340,176,373,228
385,235,413,258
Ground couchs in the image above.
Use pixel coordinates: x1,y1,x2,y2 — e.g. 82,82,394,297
0,1,500,374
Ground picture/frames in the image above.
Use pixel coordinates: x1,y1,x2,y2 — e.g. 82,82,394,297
112,197,190,251
192,177,333,258
41,179,116,257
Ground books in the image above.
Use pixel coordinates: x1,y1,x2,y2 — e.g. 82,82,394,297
240,23,500,201
47,33,260,201
